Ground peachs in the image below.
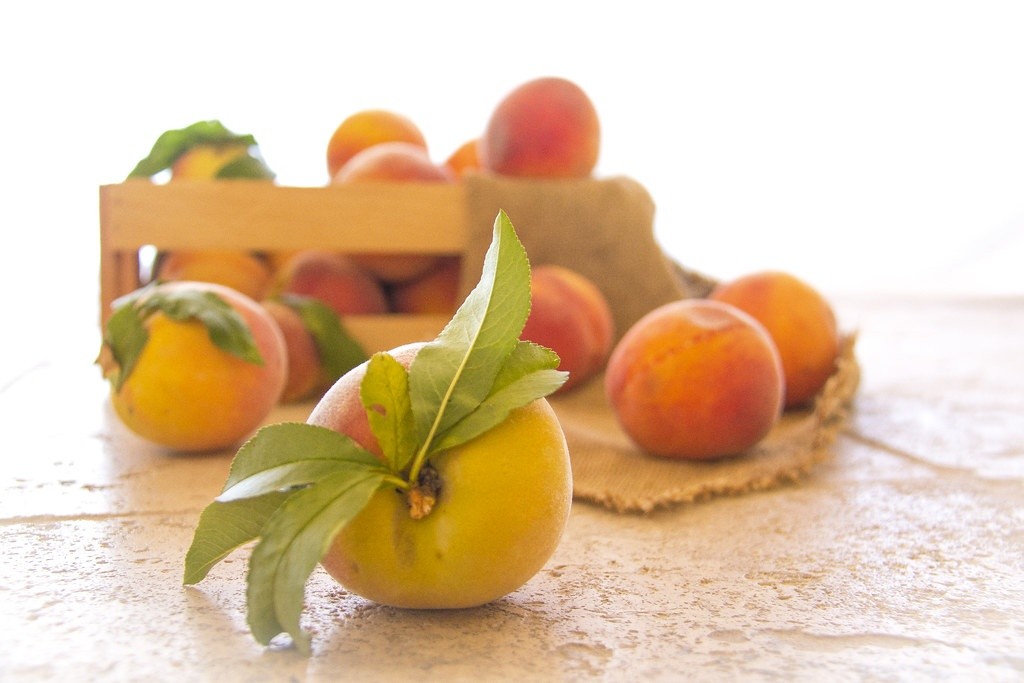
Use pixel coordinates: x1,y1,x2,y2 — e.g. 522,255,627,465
103,75,840,611
